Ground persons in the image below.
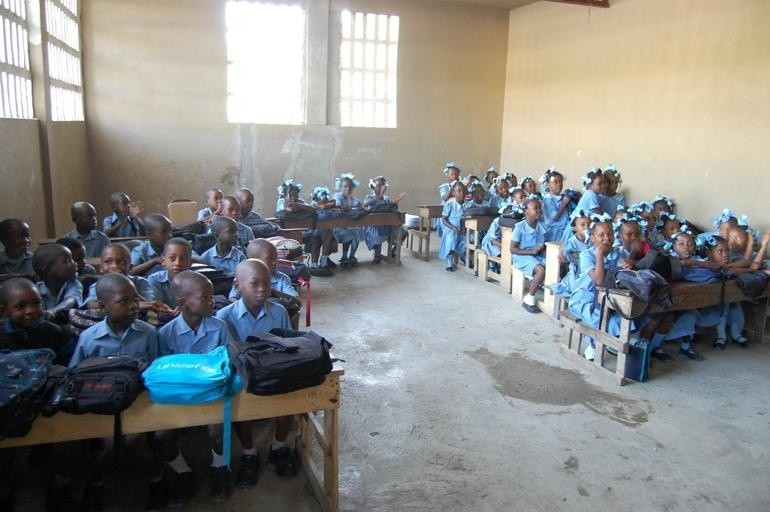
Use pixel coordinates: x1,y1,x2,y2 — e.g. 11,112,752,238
431,161,769,373
275,170,422,277
2,188,302,512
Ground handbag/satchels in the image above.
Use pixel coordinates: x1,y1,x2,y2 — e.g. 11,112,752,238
625,336,650,382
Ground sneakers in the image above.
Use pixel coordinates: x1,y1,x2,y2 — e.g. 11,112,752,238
45,484,104,511
146,442,292,512
310,257,381,276
583,343,596,361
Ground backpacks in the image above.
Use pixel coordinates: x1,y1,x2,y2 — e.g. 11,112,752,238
0,349,149,437
141,328,333,405
463,206,500,216
682,266,768,298
605,249,673,320
347,203,398,219
173,221,216,256
241,220,312,285
273,204,345,219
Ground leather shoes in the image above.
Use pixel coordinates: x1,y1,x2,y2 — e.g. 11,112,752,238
712,335,753,349
445,256,472,271
652,345,703,362
522,302,538,312
